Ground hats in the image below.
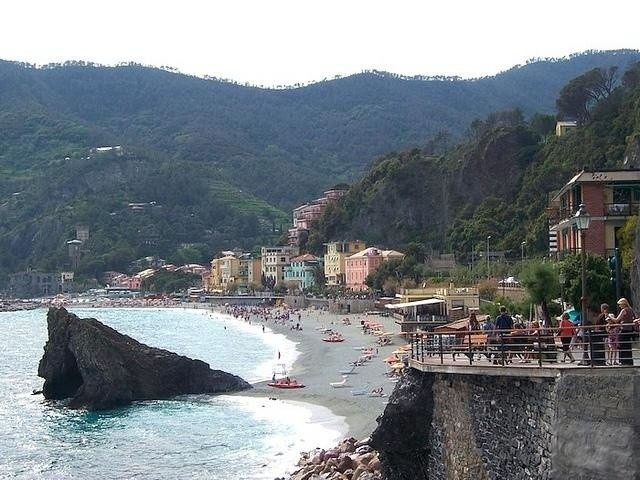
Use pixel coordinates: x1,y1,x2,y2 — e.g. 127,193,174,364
605,314,615,321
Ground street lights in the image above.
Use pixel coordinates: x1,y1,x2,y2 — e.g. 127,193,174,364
574,202,592,364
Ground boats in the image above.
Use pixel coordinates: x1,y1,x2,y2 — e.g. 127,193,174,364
267,383,305,388
323,338,345,342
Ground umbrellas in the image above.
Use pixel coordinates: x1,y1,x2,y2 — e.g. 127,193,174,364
361,319,412,369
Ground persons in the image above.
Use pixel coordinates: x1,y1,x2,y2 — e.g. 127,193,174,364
371,386,383,394
224,303,303,333
466,297,640,366
